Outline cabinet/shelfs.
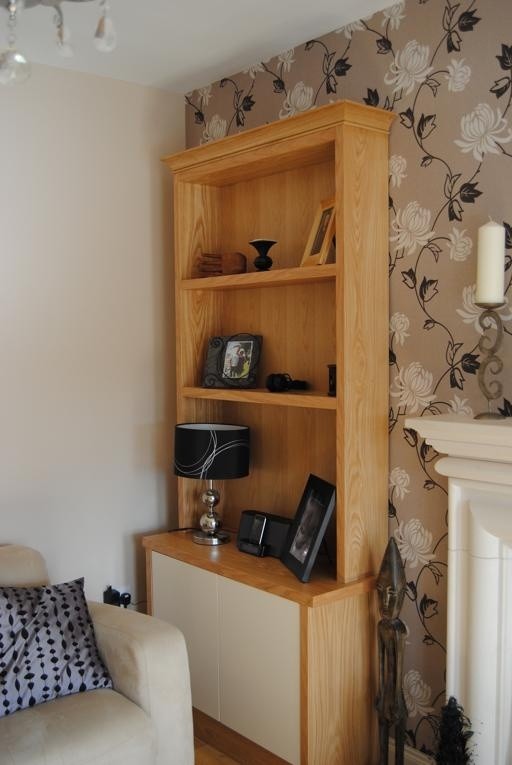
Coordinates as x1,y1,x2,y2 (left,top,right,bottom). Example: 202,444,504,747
140,531,379,765
159,98,399,586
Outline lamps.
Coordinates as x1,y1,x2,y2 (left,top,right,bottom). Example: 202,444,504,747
173,423,249,544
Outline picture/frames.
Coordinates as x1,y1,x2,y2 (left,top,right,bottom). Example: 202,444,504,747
280,474,336,583
298,199,336,265
202,332,262,389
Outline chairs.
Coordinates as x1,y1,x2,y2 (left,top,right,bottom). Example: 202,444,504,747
1,546,194,764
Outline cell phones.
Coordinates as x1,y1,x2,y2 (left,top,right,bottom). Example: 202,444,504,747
250,515,267,546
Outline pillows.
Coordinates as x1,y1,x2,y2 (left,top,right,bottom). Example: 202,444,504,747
0,578,109,719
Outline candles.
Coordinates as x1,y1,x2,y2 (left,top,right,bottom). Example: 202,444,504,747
474,214,506,304
